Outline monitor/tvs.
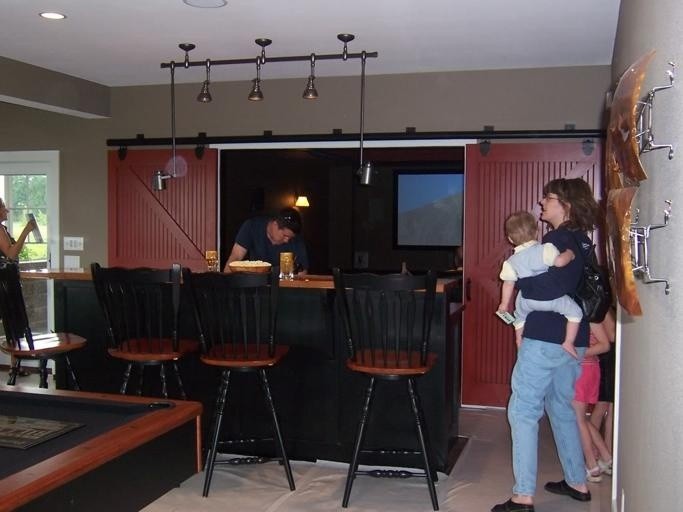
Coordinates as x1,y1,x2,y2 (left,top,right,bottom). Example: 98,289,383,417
1,415,88,450
25,213,43,244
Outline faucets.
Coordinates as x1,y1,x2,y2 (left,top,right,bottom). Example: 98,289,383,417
584,459,613,482
8,367,31,377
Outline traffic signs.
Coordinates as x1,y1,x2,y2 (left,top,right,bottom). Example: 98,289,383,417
63,236,84,251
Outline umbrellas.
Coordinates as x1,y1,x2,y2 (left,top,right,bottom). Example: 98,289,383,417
491,498,535,512
545,480,590,501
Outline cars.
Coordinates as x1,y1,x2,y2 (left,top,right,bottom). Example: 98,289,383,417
281,258,292,280
206,257,217,270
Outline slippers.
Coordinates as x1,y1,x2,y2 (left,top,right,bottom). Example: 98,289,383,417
560,229,613,322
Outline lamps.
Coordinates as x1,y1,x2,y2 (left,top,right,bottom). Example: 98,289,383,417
0,257,88,390
90,261,190,401
330,266,439,511
182,265,295,497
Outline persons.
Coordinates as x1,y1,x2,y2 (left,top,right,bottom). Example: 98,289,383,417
588,299,617,478
0,199,37,378
489,176,610,511
573,309,616,485
495,210,582,358
224,207,311,279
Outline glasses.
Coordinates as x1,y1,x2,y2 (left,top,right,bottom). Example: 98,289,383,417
153,34,379,192
105,124,608,161
295,195,311,208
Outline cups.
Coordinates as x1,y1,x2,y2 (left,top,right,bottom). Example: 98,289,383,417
545,195,559,201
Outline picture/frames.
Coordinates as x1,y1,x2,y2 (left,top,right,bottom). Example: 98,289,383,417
0,384,204,512
